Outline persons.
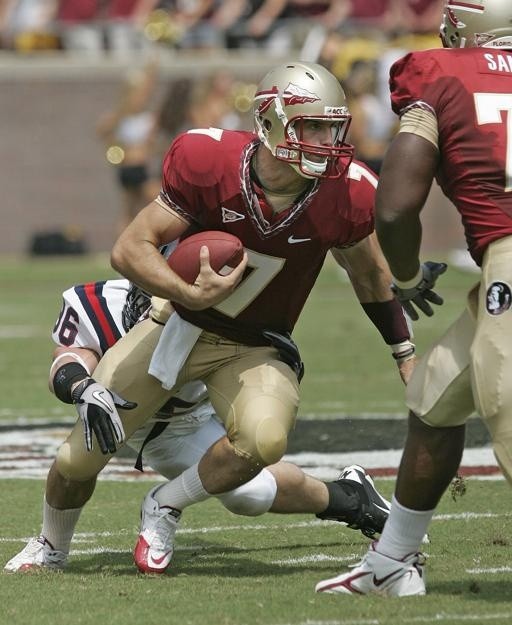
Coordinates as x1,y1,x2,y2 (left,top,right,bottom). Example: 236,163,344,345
311,1,512,598
48,239,394,542
4,59,419,574
1,0,449,227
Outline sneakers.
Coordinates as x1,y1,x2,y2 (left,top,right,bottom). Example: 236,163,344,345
338,465,431,544
3,533,69,577
314,542,428,599
132,481,183,575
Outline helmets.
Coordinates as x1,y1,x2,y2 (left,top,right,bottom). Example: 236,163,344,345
252,60,356,182
438,0,512,51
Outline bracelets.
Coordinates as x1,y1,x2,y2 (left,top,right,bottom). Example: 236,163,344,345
391,345,414,359
388,339,414,368
53,362,91,405
389,262,423,292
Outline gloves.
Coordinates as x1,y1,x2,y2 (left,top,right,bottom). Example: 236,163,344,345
389,259,449,322
69,377,139,454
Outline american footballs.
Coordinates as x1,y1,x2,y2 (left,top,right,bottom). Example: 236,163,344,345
167,230,242,286
